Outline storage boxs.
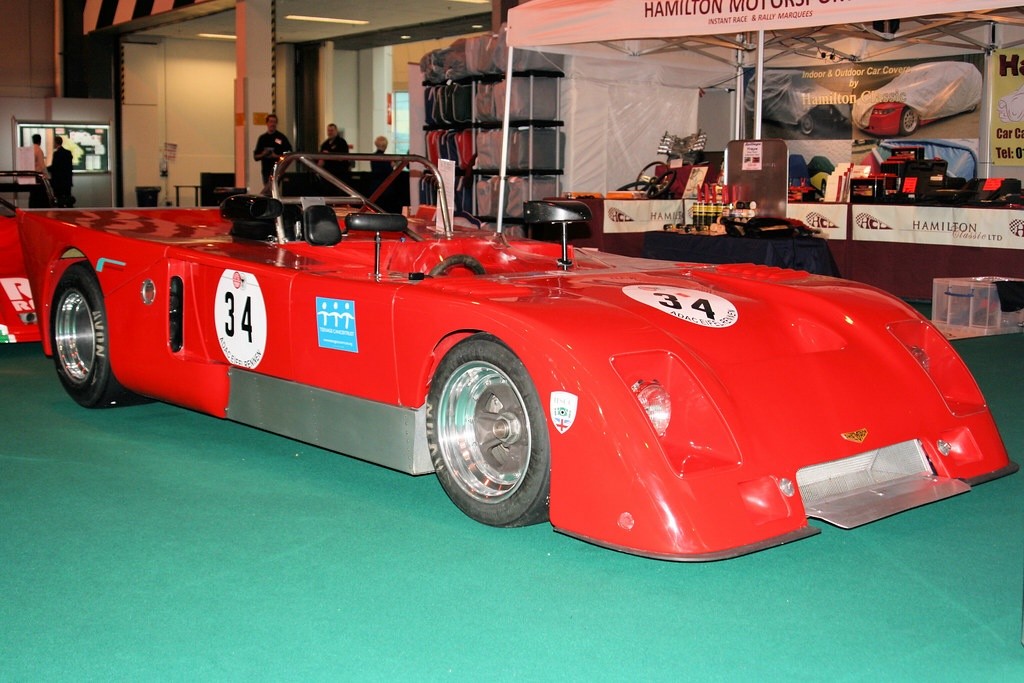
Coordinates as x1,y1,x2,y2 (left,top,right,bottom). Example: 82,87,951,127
933,276,1023,329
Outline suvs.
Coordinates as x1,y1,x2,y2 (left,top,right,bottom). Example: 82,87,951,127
745,70,850,135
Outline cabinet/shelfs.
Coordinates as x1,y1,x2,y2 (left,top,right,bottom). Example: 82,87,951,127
422,71,565,223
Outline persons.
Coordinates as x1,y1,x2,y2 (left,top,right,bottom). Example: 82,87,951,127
370,136,396,185
28,135,48,208
48,136,73,197
320,124,349,176
254,115,292,185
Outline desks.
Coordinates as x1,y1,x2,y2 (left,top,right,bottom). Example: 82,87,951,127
642,231,841,278
173,184,201,209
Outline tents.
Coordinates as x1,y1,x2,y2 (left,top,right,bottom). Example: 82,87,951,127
496,0,1024,236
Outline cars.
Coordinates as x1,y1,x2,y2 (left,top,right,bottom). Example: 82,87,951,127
0,170,65,343
853,59,983,136
14,153,1020,564
